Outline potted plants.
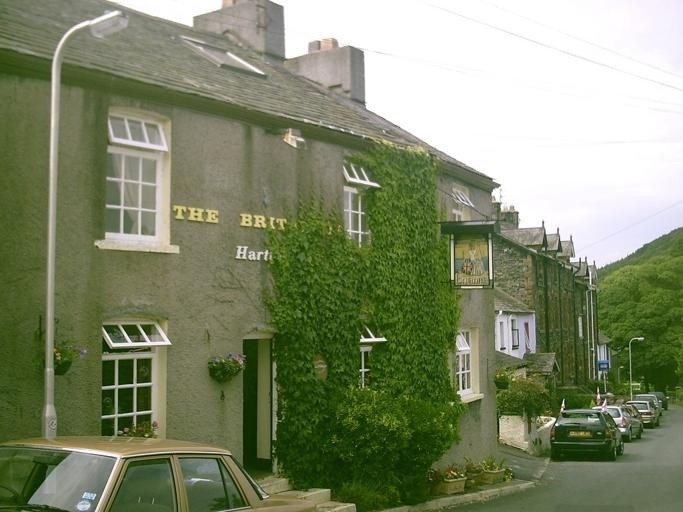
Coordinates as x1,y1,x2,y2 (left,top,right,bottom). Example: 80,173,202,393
464,456,484,483
480,454,506,484
429,466,442,496
439,462,467,495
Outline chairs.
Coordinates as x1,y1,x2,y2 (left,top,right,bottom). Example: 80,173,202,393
31,468,225,512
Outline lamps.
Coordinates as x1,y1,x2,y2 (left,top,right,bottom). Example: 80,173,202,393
265,126,307,151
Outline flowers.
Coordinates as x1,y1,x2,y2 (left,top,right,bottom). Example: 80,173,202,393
116,418,159,438
51,345,87,369
208,353,245,382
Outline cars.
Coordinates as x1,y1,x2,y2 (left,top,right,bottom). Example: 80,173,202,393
0,435,317,512
550,392,670,461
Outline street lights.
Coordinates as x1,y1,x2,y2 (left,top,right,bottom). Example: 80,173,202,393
42,10,129,437
629,337,644,401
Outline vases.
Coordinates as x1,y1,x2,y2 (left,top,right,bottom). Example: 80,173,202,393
42,359,72,376
